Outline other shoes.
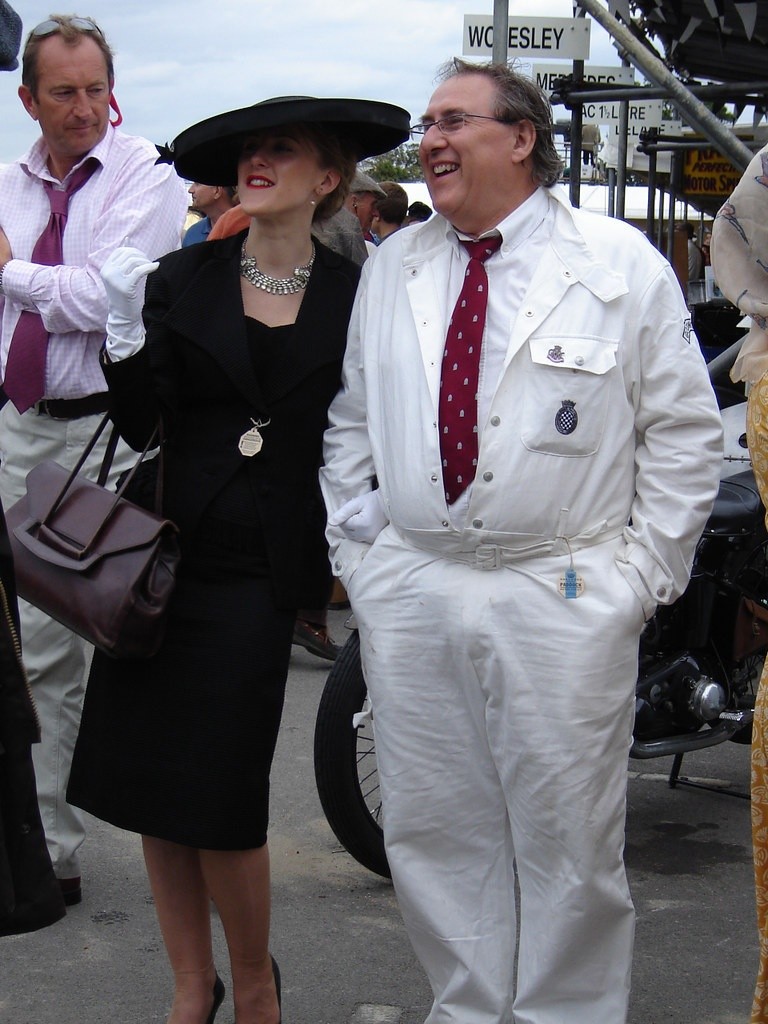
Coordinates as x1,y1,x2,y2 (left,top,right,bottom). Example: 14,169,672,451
57,876,82,905
292,620,344,660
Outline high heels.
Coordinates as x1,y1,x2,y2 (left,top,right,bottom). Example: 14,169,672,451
270,953,282,1024
205,973,225,1024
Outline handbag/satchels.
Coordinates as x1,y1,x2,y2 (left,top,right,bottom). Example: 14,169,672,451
5,398,187,666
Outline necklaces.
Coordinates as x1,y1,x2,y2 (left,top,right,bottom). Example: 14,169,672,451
242,235,314,295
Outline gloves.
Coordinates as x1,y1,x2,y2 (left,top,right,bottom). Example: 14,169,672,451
326,487,389,545
96,236,160,363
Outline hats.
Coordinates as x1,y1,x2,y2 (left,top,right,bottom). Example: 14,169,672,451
350,172,387,201
153,95,412,188
0,0,22,71
675,222,697,238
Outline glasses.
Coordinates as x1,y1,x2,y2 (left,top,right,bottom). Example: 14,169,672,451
22,18,109,85
407,114,518,142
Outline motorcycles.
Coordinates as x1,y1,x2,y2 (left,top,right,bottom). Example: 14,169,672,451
313,299,768,879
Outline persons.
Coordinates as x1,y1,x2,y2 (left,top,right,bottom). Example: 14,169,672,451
57,90,410,1024
0,18,187,905
317,60,725,1024
180,170,432,660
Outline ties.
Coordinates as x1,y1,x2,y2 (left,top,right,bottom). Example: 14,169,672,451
3,157,101,415
438,233,503,506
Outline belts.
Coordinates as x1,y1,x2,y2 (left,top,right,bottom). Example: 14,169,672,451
31,391,110,418
